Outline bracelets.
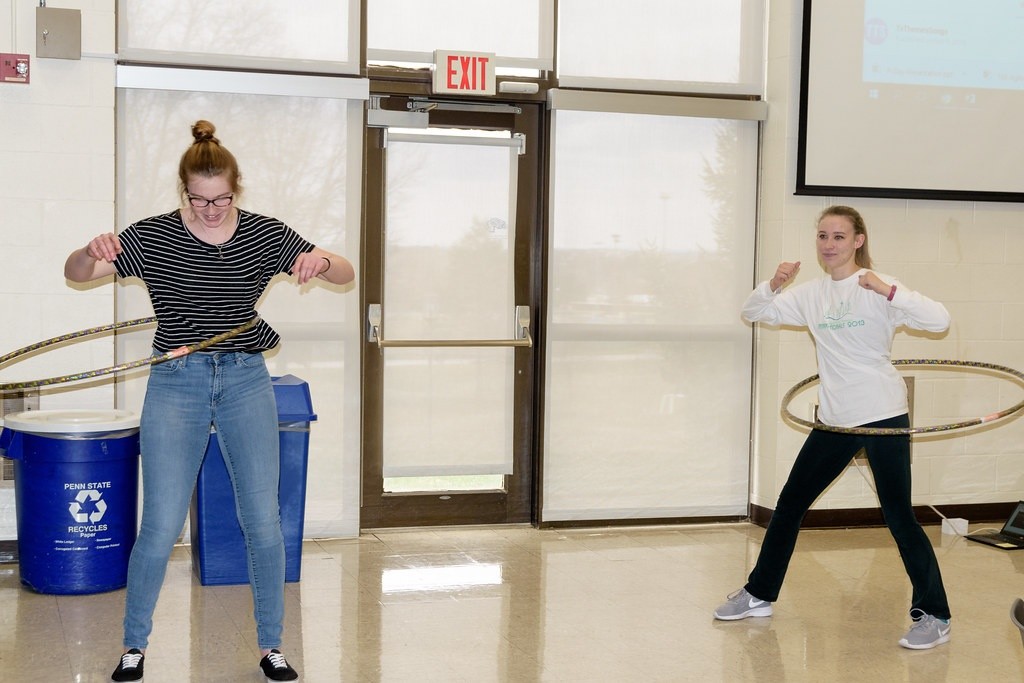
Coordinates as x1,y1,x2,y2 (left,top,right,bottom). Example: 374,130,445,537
318,257,331,274
886,284,897,301
771,281,776,290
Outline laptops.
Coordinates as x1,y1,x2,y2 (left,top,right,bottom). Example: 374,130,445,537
963,501,1024,550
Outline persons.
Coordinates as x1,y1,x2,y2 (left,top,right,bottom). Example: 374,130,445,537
713,207,953,650
65,121,355,682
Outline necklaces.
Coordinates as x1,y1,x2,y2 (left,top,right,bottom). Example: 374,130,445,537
196,218,232,259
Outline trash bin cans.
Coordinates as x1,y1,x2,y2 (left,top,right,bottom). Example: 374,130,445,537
192,371,319,587
0,408,143,596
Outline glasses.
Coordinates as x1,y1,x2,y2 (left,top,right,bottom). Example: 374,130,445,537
186,188,234,207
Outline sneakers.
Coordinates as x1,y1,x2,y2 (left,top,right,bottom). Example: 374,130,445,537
898,608,951,649
111,648,145,683
714,588,773,620
258,649,299,683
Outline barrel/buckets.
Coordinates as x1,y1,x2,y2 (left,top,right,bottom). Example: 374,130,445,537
0,409,140,597
